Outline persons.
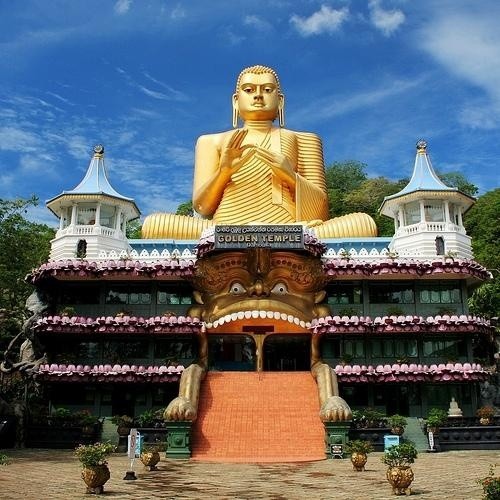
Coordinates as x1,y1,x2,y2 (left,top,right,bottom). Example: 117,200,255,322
140,66,380,240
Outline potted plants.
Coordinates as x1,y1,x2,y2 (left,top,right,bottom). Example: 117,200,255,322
389,414,408,435
73,442,117,494
426,407,447,433
381,444,418,495
478,407,494,425
141,446,160,471
347,439,369,472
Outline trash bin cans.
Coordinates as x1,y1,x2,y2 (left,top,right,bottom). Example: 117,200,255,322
383,435,401,458
128,431,144,458
